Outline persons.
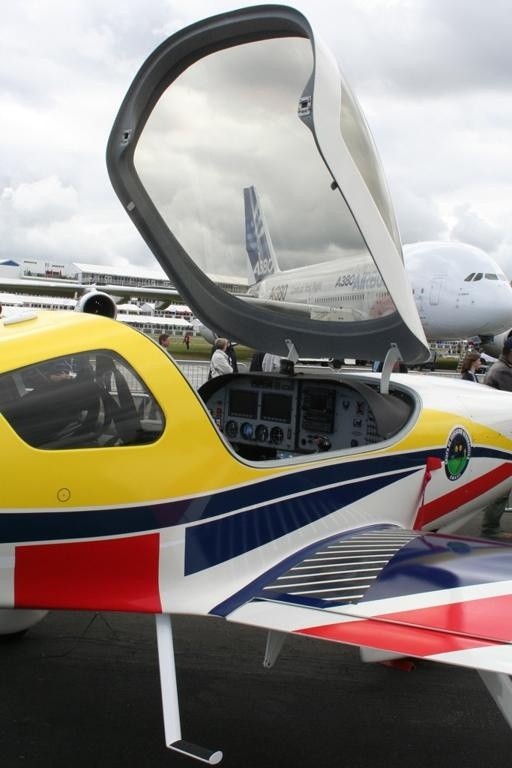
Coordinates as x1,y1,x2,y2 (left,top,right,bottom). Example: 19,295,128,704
96,355,112,391
159,334,265,378
372,330,512,391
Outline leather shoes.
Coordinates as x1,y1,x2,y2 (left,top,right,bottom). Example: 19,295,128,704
480,530,512,539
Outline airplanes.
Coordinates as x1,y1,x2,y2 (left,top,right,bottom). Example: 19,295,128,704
0,184,512,376
0,0,511,768
